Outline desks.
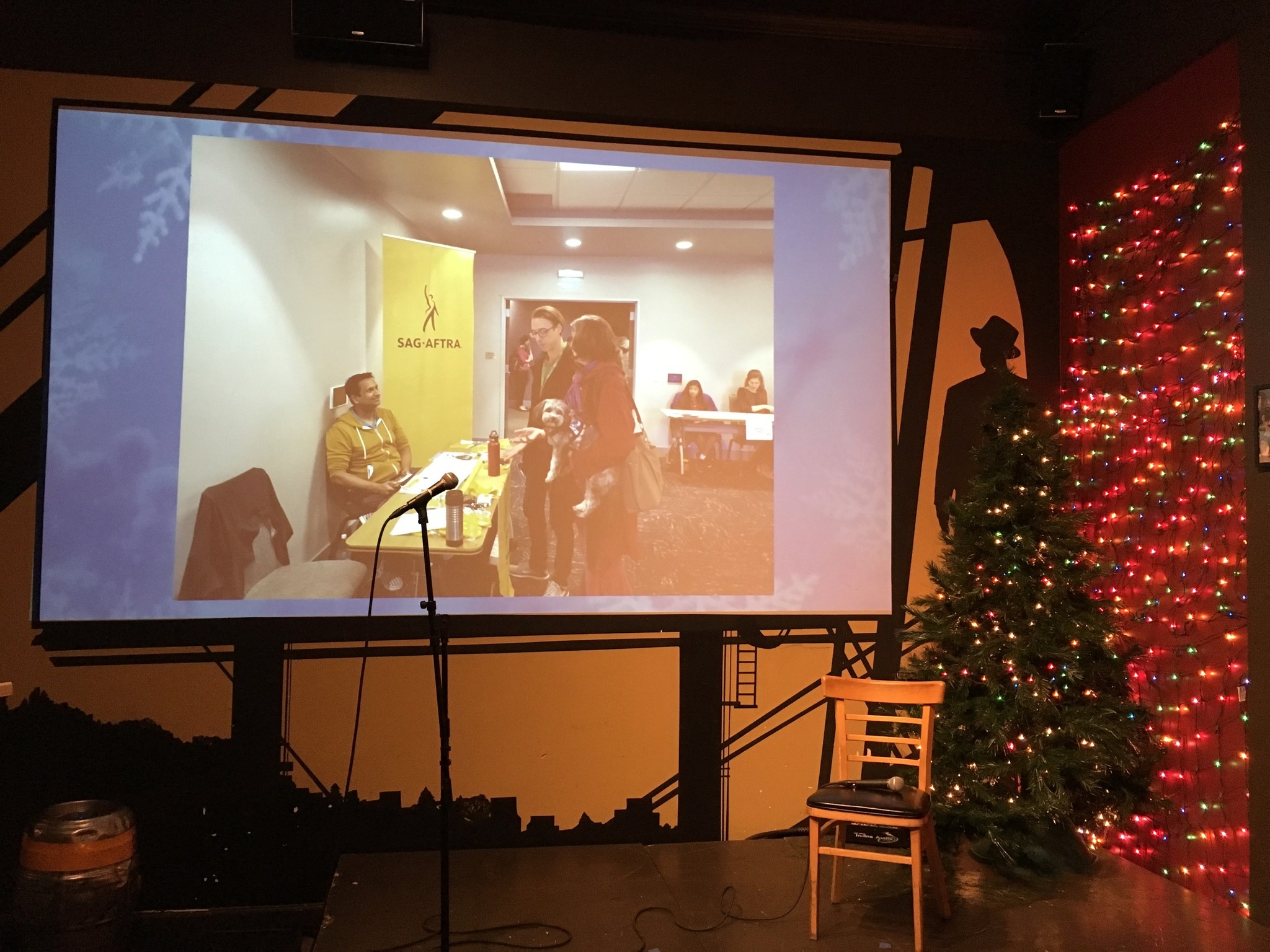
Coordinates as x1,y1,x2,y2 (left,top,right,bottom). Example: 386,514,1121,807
666,407,774,479
346,439,517,596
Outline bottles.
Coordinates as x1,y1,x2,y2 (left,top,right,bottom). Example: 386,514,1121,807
488,430,500,476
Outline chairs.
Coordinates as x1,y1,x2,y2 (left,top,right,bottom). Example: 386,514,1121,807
671,392,753,467
203,470,367,598
805,675,951,952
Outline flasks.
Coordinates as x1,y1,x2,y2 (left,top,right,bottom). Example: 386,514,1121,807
446,490,463,546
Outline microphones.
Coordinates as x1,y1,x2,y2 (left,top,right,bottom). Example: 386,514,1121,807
390,473,458,520
837,776,904,792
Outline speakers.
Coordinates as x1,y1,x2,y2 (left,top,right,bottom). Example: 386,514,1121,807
291,0,425,52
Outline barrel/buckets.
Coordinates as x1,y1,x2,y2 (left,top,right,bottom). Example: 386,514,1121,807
19,799,137,929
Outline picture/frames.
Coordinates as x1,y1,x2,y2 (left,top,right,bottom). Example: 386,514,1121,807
1253,383,1270,471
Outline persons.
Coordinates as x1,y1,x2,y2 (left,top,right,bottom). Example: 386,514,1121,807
613,326,630,378
507,305,577,596
516,335,534,412
510,314,639,596
324,372,416,590
668,379,715,474
736,367,774,480
